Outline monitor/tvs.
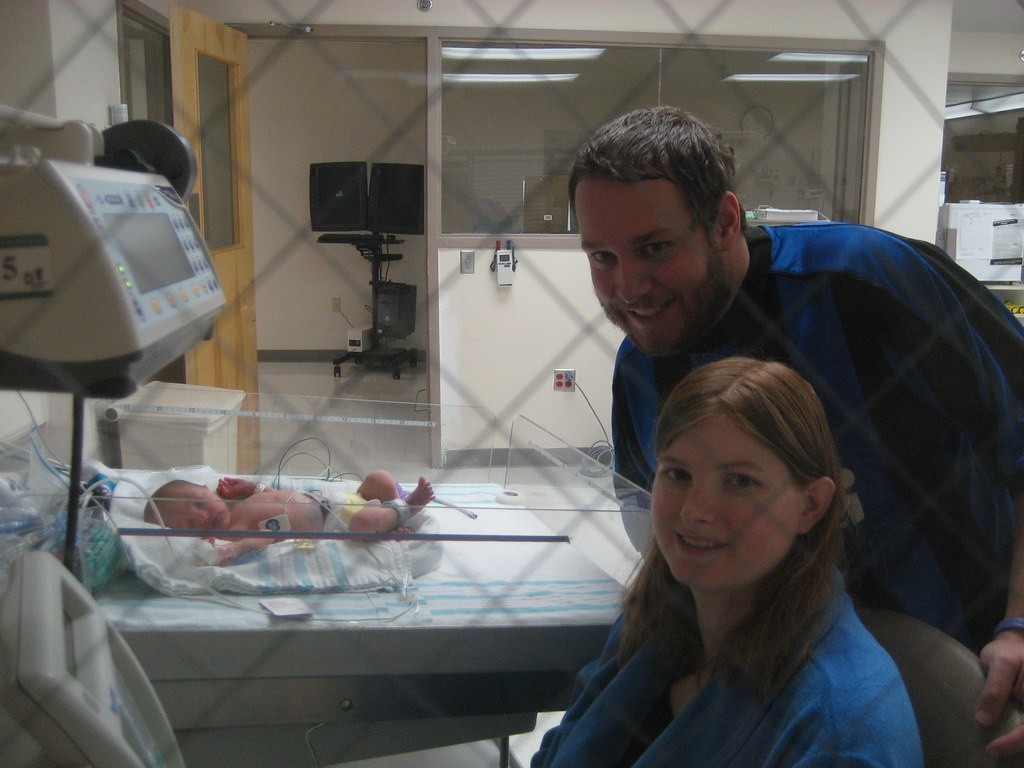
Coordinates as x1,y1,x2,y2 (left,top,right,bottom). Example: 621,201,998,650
309,161,368,232
366,163,425,234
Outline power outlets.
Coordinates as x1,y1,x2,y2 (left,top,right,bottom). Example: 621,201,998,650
554,369,576,392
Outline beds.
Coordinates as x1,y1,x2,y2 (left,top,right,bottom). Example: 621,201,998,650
0,422,655,768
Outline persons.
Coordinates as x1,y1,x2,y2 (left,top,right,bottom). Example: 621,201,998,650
143,470,436,569
525,353,923,768
565,104,1024,755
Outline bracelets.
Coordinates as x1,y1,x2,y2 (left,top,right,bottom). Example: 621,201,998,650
991,615,1024,640
